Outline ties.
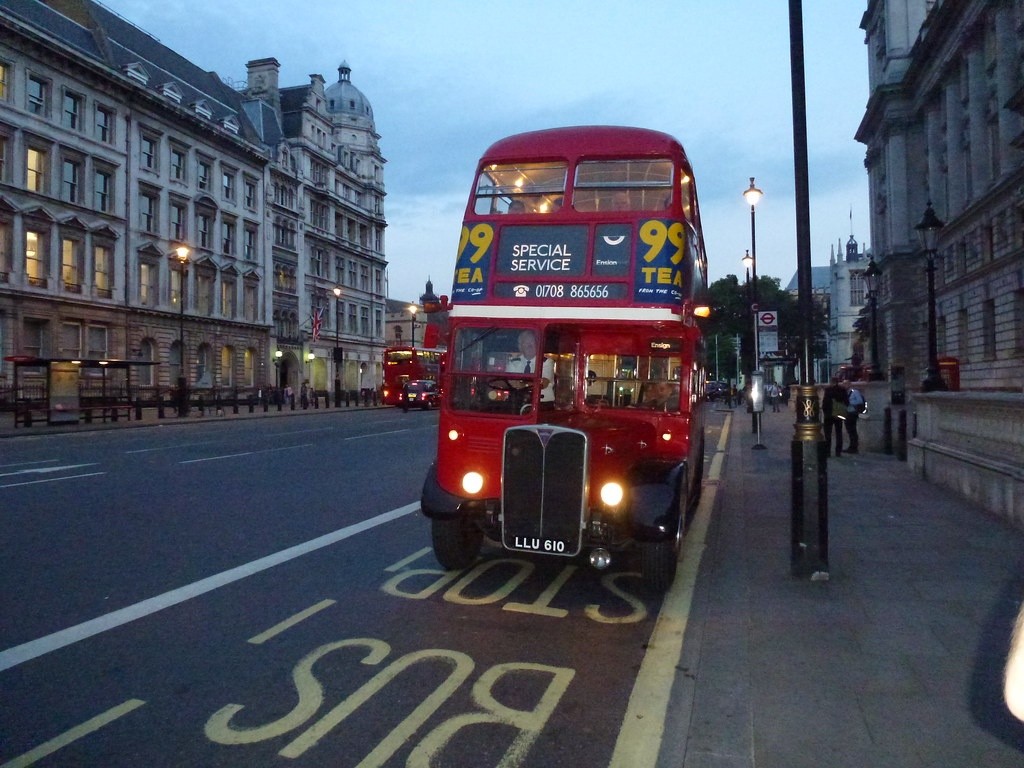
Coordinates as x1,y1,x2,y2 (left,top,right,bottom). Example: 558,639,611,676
524,361,531,374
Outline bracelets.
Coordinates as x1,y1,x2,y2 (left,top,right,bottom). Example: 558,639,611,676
525,381,529,388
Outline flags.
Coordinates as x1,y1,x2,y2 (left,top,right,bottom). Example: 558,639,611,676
310,310,322,342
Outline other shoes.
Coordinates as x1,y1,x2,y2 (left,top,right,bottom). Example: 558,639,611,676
836,452,840,457
842,447,858,454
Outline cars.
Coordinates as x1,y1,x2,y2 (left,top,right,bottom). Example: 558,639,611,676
705,380,730,400
398,380,441,411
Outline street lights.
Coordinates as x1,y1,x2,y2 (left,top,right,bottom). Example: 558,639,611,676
740,177,765,450
333,286,344,408
174,243,190,419
409,301,419,348
861,255,884,381
914,197,949,392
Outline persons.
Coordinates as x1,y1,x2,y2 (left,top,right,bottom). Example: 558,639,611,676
965,571,1024,756
507,201,525,214
626,380,680,411
486,327,557,416
768,383,783,413
300,379,313,406
819,378,850,457
283,383,292,407
612,191,631,210
841,379,864,454
731,383,752,407
551,196,564,212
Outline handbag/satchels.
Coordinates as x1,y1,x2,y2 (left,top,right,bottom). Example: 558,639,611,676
848,389,866,412
831,398,848,421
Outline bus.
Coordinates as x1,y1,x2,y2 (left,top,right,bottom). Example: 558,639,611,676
419,125,724,592
381,345,451,408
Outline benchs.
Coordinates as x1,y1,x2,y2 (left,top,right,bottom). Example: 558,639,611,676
24,402,132,428
